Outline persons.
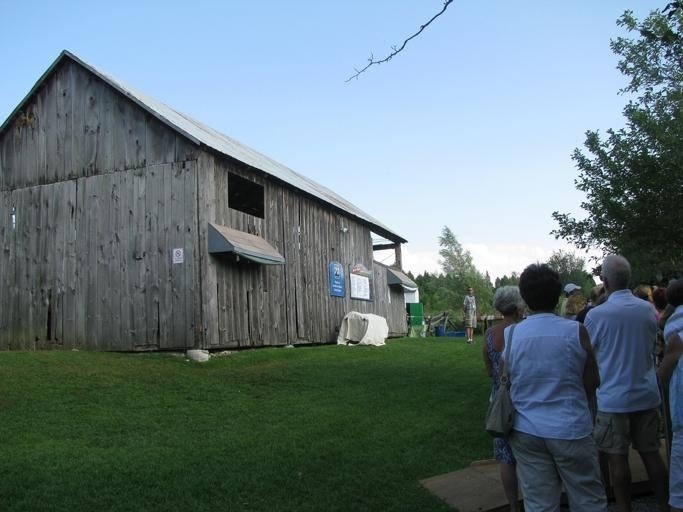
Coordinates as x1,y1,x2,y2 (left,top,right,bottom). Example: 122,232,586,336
463,287,477,345
581,254,670,511
554,277,683,441
480,286,528,512
500,261,611,512
656,277,683,511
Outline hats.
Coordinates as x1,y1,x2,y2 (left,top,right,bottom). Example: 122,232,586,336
563,283,581,298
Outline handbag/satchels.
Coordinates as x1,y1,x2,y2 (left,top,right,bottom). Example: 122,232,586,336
486,383,514,437
